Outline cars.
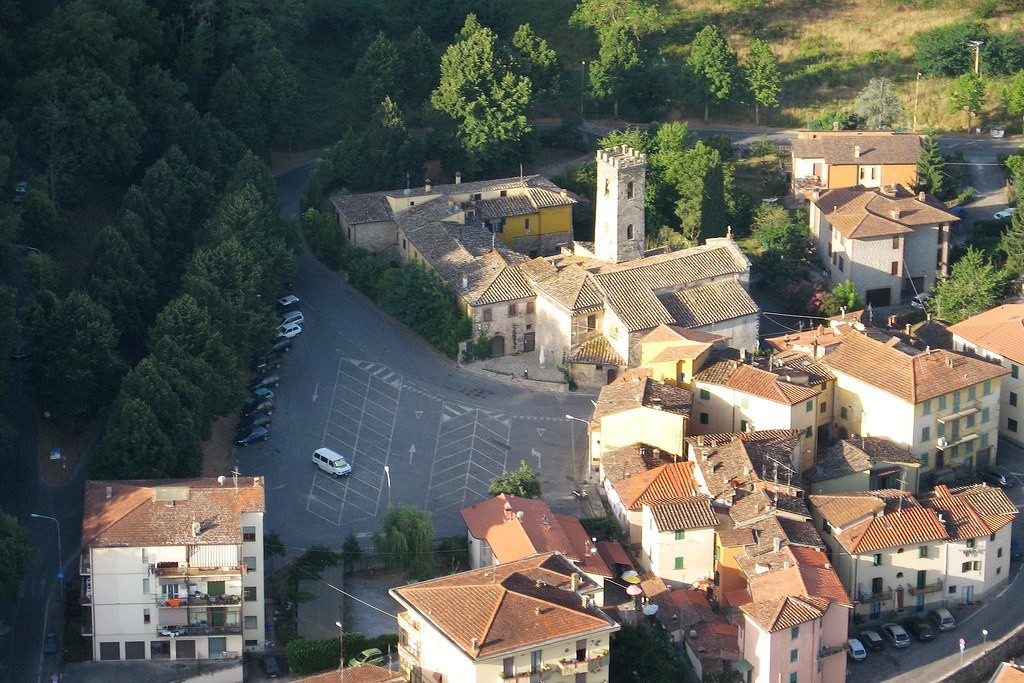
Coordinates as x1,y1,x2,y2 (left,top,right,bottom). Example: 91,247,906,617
348,648,385,671
908,619,936,643
241,335,293,418
949,206,968,220
233,425,270,447
910,292,933,311
276,323,303,341
45,633,58,655
861,630,884,652
880,622,911,649
1011,537,1024,560
993,208,1017,220
236,411,272,432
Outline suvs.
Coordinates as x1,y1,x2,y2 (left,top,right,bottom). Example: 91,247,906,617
276,310,305,328
929,607,956,633
978,466,1015,489
272,295,300,314
847,638,866,663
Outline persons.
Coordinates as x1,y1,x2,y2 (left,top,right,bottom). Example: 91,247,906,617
524,366,528,379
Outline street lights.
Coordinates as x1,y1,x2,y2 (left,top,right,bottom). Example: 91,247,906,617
336,620,344,671
30,512,66,604
566,415,591,476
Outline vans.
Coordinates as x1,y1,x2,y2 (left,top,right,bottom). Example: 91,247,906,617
990,124,1007,139
312,448,352,480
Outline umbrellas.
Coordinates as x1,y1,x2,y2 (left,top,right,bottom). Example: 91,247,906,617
621,570,642,584
626,584,642,595
643,603,659,615
538,344,545,365
